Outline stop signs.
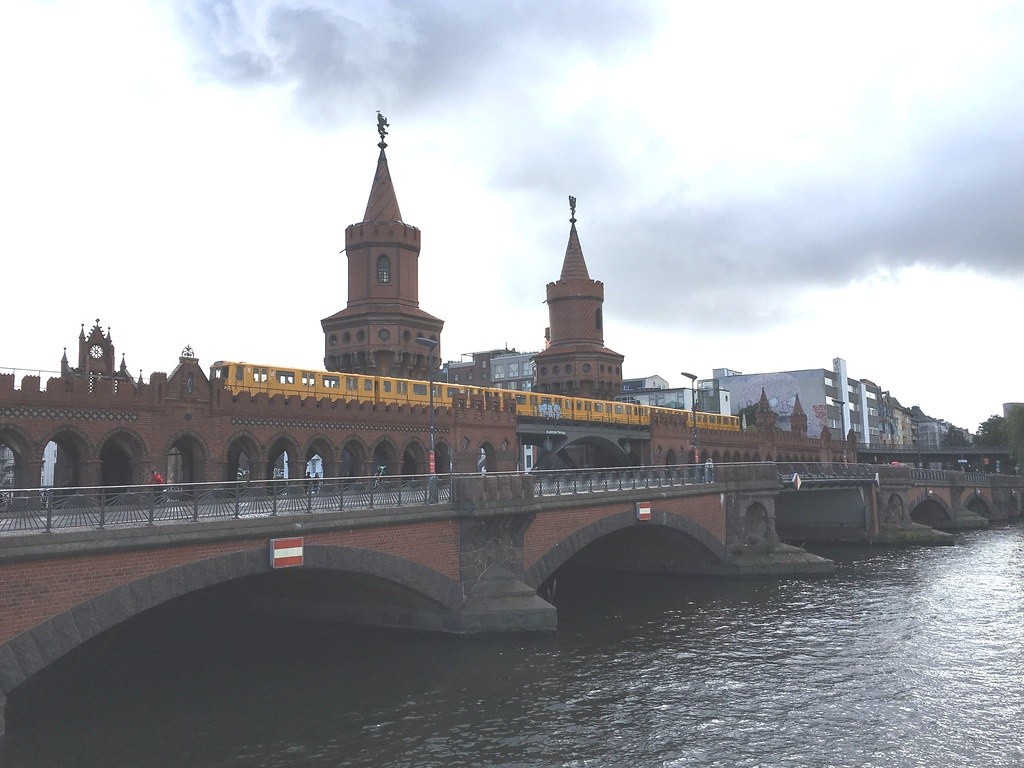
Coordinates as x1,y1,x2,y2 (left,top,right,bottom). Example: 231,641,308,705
270,537,304,569
637,502,652,520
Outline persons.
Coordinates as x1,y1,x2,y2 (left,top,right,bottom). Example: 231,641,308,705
314,473,319,478
305,472,311,478
374,466,385,487
705,458,713,483
146,469,164,504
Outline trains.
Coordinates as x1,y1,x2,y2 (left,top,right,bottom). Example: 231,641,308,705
209,360,741,433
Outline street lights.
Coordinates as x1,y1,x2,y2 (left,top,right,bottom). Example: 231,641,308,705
680,372,700,464
414,338,437,474
831,399,847,462
